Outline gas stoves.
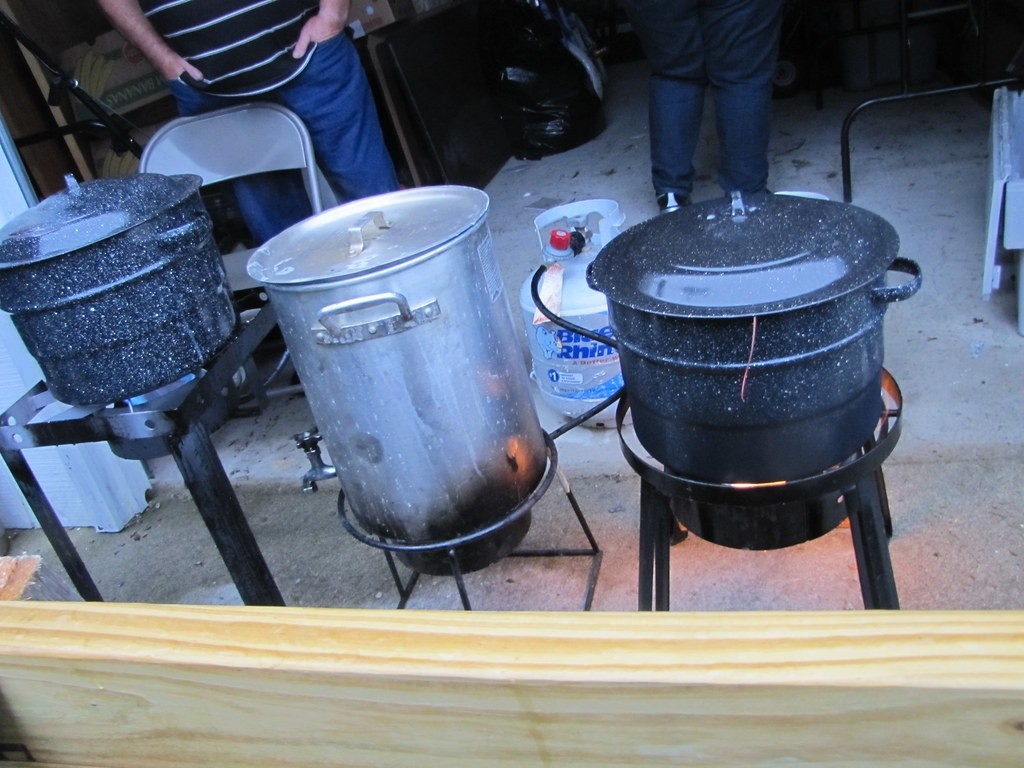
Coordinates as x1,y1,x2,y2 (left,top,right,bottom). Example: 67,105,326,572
0,284,296,606
614,365,904,614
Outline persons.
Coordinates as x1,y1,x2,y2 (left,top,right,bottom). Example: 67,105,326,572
612,1,785,222
100,0,404,397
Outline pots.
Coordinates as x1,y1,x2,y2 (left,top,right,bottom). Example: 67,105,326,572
583,188,924,482
0,172,240,406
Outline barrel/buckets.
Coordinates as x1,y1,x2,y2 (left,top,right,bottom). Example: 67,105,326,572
246,181,553,551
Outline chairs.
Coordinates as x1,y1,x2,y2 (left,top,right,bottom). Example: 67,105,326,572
140,106,335,401
